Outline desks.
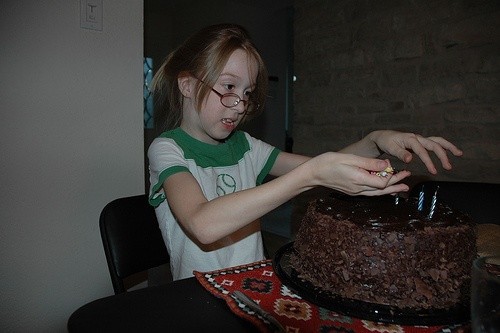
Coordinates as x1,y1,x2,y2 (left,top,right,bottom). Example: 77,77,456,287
67,222,500,333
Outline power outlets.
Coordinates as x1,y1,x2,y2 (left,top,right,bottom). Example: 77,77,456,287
79,0,103,31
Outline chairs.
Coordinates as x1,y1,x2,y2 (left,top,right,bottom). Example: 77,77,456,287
99,194,173,296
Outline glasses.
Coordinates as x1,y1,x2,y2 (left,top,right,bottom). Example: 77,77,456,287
195,75,261,116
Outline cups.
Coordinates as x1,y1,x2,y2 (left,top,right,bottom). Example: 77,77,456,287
471,255,500,333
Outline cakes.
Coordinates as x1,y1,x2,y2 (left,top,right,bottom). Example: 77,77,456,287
286,190,477,311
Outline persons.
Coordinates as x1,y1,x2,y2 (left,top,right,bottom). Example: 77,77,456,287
147,25,462,281
